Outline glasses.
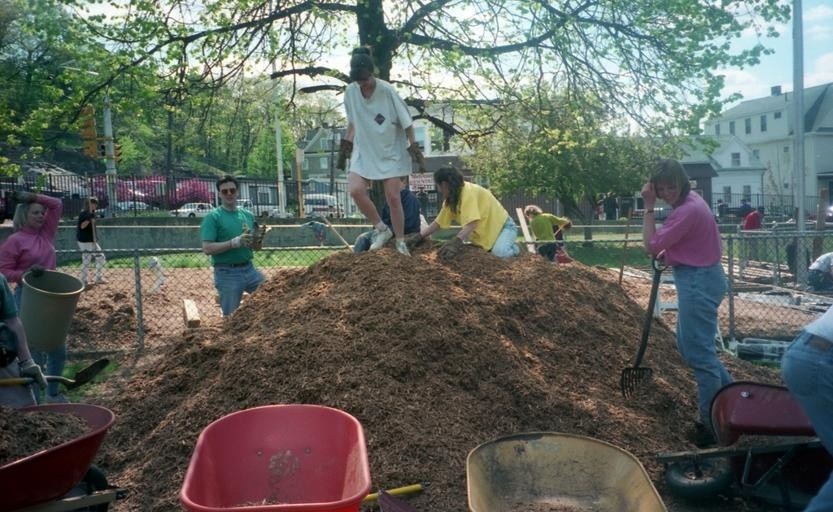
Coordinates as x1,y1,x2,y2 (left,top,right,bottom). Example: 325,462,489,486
221,188,236,195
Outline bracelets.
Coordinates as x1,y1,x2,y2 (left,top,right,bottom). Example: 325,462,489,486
643,208,654,215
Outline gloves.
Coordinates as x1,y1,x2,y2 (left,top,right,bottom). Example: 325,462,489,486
335,139,353,170
19,358,48,390
436,236,463,260
407,142,425,173
28,263,44,276
406,232,422,249
253,221,266,250
5,191,36,203
231,229,255,248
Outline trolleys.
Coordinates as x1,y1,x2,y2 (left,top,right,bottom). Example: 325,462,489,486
1,403,119,510
463,430,673,512
177,402,371,512
652,381,831,509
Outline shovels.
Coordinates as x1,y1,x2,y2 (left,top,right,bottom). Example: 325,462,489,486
621,255,668,404
1,356,110,391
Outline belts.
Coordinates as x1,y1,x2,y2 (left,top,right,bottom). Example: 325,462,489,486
810,335,832,350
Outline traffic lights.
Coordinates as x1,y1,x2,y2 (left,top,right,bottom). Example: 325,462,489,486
114,143,122,163
100,143,105,157
78,102,96,157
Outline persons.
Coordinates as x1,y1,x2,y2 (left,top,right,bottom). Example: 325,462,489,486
785,234,810,276
407,166,521,258
782,303,833,512
524,205,570,261
353,177,421,254
0,187,69,403
596,192,619,221
77,197,109,287
534,216,572,250
808,250,833,292
0,272,48,407
641,159,733,431
336,47,425,256
718,197,765,261
200,175,265,316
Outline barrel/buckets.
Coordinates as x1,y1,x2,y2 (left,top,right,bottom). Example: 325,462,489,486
18,271,85,355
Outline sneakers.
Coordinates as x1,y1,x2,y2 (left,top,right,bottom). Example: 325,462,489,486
370,226,393,250
395,240,411,257
47,393,70,402
96,280,107,283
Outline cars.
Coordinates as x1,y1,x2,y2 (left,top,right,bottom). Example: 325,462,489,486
632,202,832,225
